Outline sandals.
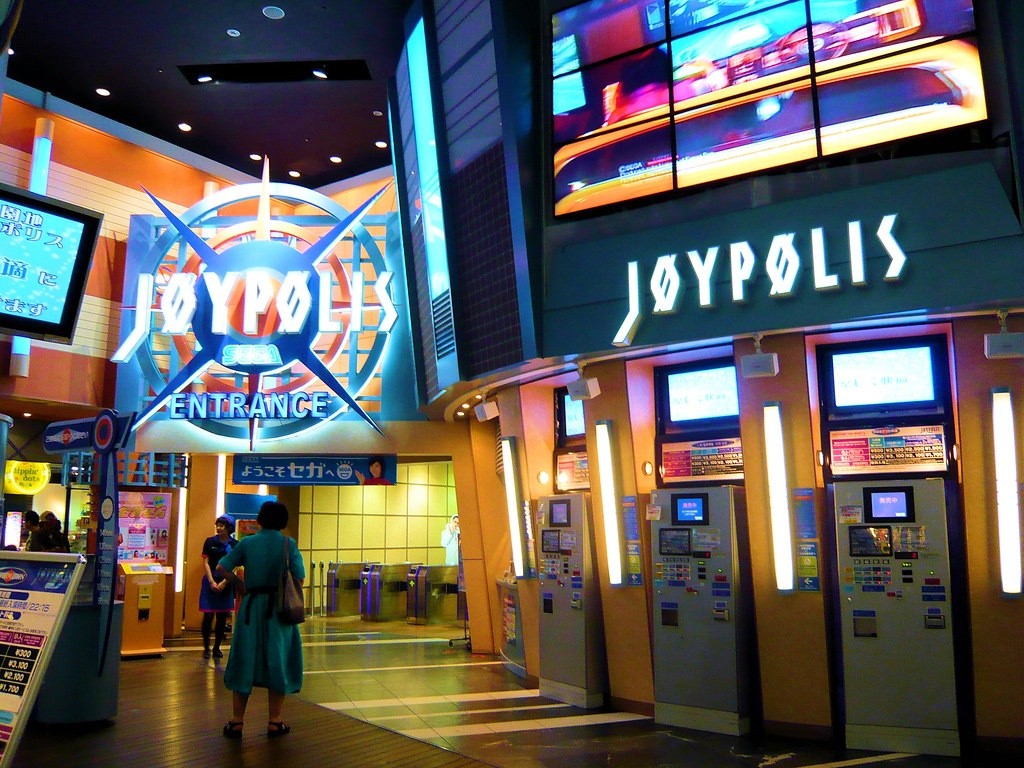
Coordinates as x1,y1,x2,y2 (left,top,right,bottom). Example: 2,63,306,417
266,721,290,737
223,721,243,738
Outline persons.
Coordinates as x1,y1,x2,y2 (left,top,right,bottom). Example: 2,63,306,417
216,500,306,743
197,513,240,659
441,515,461,566
21,510,72,553
354,457,394,484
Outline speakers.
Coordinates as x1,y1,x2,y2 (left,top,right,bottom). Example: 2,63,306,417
474,400,500,423
567,377,601,401
742,353,780,378
984,332,1024,359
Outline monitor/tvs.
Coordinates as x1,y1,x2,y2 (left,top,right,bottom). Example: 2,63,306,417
829,345,943,412
664,363,739,425
870,492,907,517
562,394,586,437
0,183,105,346
552,503,567,523
676,499,703,520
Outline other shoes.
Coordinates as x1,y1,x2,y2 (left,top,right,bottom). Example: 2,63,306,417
213,649,223,657
204,650,210,658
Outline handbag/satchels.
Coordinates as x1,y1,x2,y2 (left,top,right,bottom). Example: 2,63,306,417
277,541,305,624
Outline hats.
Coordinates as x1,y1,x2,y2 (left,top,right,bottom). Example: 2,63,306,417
219,514,235,535
39,511,57,522
